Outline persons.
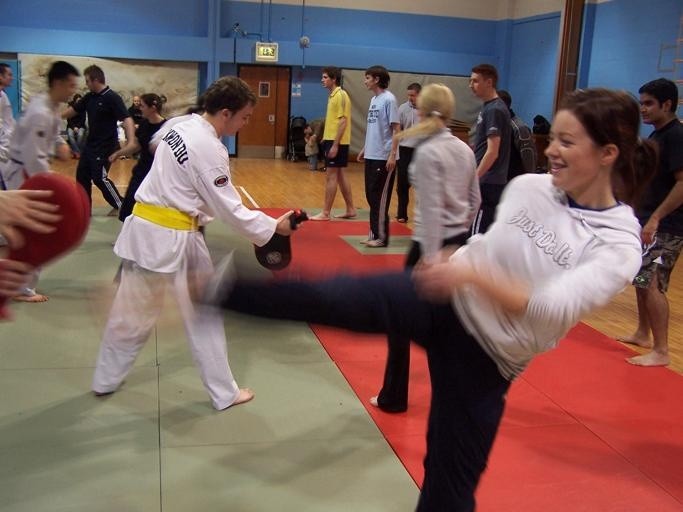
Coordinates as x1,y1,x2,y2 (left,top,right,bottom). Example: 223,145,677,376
304,64,535,244
91,76,305,413
369,82,482,412
617,78,683,367
186,87,661,512
0,59,170,323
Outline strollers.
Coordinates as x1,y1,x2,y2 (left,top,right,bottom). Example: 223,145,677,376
285,115,309,162
